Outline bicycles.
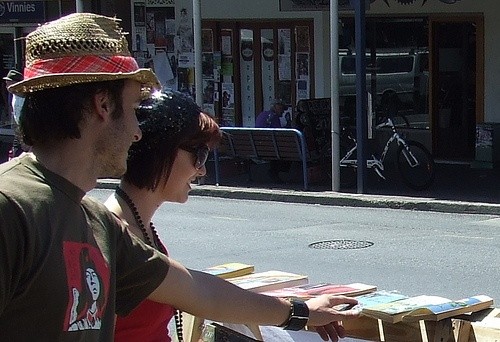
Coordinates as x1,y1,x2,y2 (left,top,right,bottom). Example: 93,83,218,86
340,114,435,191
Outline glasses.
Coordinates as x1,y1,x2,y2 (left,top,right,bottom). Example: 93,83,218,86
178,144,210,169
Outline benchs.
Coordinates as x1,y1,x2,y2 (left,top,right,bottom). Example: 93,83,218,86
214,127,316,190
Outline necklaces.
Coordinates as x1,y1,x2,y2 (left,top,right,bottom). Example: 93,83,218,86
113,184,186,341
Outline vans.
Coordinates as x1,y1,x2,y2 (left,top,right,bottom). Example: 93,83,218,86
338,48,429,114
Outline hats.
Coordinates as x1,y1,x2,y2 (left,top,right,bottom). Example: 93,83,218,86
3,70,24,82
126,88,201,161
270,98,288,110
7,12,162,98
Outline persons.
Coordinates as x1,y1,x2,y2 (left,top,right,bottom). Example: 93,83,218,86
3,70,28,160
254,102,288,129
0,11,362,341
96,88,226,342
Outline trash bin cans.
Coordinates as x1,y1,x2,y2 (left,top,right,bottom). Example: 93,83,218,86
0,124,15,164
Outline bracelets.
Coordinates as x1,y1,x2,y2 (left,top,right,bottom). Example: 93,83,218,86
277,297,311,333
